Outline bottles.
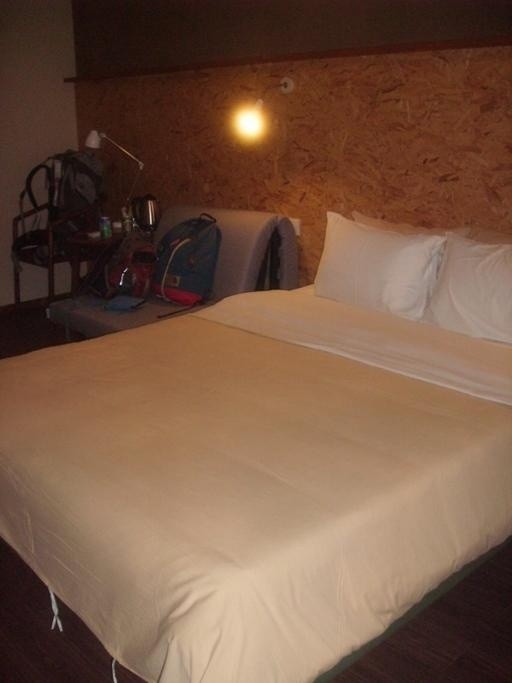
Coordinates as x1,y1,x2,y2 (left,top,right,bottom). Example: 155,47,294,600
121,195,133,230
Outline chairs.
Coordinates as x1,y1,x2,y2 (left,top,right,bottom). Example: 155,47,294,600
11,148,100,314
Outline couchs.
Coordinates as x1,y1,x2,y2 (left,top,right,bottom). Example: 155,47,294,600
48,205,300,339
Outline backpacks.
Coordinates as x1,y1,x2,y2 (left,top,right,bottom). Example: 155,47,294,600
24,148,103,232
79,213,222,318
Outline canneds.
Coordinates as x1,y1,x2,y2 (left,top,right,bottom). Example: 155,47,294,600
122,216,133,238
100,217,112,239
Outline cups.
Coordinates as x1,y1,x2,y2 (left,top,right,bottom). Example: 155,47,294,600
132,250,157,294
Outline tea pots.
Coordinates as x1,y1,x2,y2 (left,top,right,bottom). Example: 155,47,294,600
134,194,160,230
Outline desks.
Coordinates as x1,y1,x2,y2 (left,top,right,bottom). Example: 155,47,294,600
62,227,122,294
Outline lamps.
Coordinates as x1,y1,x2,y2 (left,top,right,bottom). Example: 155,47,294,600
232,74,298,144
83,127,144,230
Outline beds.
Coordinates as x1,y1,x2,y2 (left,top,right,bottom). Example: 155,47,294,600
0,281,512,683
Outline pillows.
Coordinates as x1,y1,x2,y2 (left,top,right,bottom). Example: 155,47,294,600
349,207,466,238
310,209,445,323
439,229,512,345
469,224,512,244
